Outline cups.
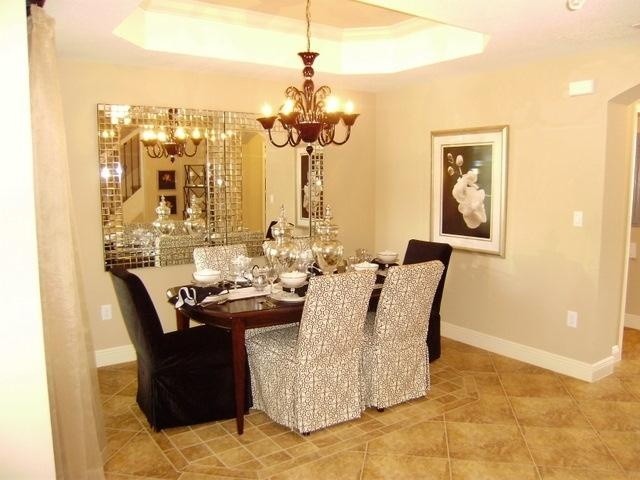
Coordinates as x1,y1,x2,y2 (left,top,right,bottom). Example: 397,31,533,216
346,248,375,266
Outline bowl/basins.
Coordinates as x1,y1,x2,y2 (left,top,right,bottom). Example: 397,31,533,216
193,269,221,283
355,262,379,272
279,272,308,286
378,252,399,261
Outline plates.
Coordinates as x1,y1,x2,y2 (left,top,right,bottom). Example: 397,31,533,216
375,259,400,265
271,292,306,304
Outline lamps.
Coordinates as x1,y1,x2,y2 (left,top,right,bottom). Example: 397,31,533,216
140,120,205,164
258,1,360,152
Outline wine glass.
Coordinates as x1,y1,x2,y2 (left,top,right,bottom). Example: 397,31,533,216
229,255,277,293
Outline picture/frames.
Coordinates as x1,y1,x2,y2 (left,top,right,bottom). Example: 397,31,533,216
156,169,178,215
294,147,323,227
428,123,510,259
